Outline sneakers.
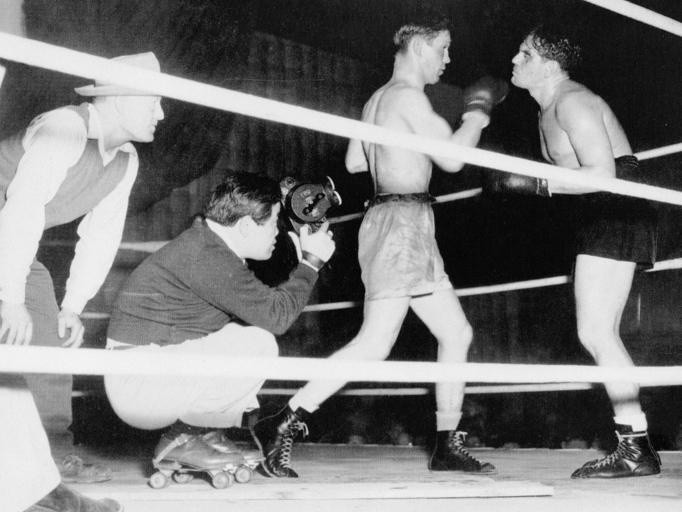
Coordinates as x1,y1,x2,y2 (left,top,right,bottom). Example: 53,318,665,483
249,405,300,477
571,422,661,479
428,430,497,475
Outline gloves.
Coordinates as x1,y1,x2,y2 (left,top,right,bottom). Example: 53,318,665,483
460,76,510,127
478,171,551,208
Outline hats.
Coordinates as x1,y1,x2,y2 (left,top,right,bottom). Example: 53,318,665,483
73,51,160,98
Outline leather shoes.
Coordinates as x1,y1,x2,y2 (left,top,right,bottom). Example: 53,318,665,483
29,483,123,511
55,457,112,483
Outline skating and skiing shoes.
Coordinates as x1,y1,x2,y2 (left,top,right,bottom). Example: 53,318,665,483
149,435,244,489
198,430,266,482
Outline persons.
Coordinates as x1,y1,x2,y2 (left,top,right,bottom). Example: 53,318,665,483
250,6,509,481
0,51,166,488
483,23,663,477
102,172,336,485
0,366,116,510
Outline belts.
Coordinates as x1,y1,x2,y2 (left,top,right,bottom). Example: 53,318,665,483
377,191,436,202
